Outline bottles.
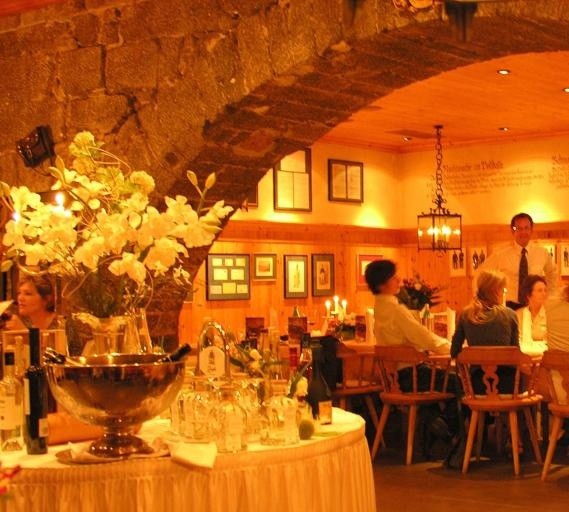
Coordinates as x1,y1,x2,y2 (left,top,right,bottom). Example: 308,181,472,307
307,340,333,426
196,320,310,379
293,264,300,288
355,309,376,343
151,342,193,363
44,344,85,366
422,303,433,333
320,263,325,283
292,305,300,317
168,375,314,455
0,339,46,455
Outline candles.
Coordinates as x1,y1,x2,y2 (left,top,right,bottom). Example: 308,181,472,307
325,301,330,319
342,300,347,315
333,295,339,312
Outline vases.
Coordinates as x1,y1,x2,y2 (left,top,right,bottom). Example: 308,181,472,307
71,312,154,357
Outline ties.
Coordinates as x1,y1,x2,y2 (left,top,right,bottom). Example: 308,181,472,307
518,248,528,302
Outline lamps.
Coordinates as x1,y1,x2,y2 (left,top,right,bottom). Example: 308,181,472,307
416,125,463,252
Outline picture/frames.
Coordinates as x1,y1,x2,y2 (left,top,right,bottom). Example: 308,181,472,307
328,158,363,202
274,146,312,211
205,253,250,301
253,253,278,279
248,186,258,207
311,253,335,297
283,254,308,299
356,252,385,287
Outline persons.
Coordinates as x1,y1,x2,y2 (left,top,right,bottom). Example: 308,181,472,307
365,260,467,457
450,213,569,460
1,275,67,361
319,265,326,282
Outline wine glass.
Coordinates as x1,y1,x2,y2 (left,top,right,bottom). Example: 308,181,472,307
43,352,187,459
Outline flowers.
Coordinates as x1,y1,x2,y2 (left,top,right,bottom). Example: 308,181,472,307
0,128,235,357
393,269,445,310
